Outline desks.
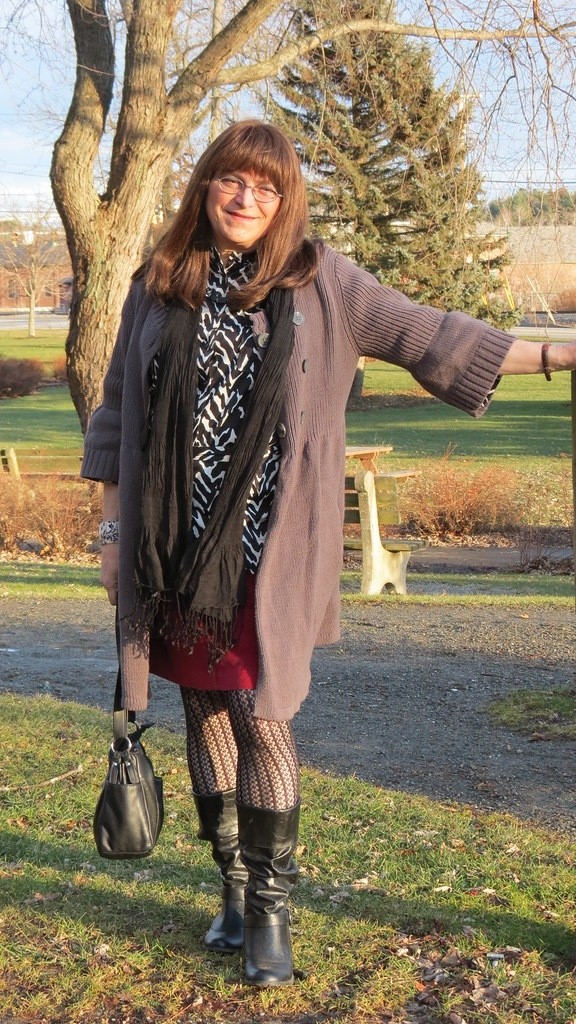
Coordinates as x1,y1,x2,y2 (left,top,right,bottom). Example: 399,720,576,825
345,446,393,474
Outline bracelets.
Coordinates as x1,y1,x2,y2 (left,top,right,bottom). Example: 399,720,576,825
98,521,119,545
542,343,552,381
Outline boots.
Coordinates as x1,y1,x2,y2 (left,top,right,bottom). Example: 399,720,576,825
191,788,249,953
233,795,302,985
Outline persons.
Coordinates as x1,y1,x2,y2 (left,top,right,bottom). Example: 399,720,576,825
79,120,576,987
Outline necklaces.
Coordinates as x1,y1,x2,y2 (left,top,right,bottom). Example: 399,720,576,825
228,272,244,286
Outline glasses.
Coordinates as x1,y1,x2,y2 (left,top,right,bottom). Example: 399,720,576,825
212,176,284,203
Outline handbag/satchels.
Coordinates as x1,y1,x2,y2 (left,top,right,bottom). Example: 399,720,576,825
93,590,164,860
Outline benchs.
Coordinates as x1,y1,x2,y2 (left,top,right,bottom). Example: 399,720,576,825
0,445,100,498
341,470,422,595
